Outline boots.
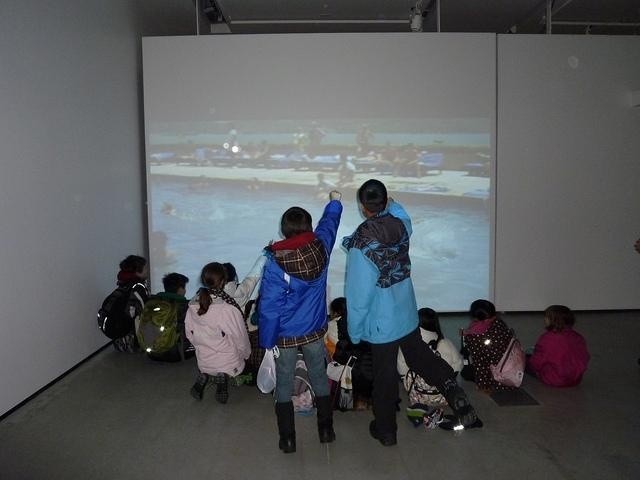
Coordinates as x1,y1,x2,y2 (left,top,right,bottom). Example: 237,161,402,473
437,375,482,430
275,400,296,453
370,393,397,445
315,396,335,442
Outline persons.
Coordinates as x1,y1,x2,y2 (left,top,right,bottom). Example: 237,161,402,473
323,296,346,364
397,299,515,426
524,305,589,387
257,192,344,453
339,180,484,447
111,240,275,403
153,119,427,193
461,144,486,174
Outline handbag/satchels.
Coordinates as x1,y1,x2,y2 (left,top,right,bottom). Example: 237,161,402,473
327,361,353,411
490,337,527,387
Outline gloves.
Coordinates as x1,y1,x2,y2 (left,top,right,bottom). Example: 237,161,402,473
424,407,443,429
406,403,428,426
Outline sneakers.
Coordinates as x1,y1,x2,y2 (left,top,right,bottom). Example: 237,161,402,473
215,373,230,404
191,373,212,400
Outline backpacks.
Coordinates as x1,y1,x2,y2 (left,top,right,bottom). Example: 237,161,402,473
401,340,449,407
137,291,183,353
273,351,318,416
97,282,146,339
